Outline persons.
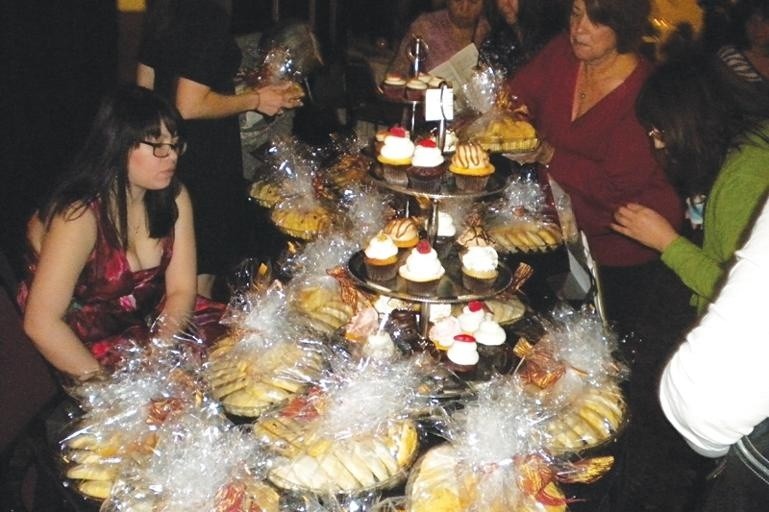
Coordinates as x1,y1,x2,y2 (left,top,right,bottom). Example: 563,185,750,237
14,85,236,384
235,17,324,272
135,0,305,300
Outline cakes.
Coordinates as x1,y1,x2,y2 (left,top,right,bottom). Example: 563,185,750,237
381,70,446,101
363,210,507,382
374,123,496,194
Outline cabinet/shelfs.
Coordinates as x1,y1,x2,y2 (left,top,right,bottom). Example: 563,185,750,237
346,31,523,402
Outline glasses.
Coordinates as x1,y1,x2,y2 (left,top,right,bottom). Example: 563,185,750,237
142,139,187,158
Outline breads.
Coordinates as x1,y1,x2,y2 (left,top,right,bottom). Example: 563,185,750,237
66,435,117,499
207,345,398,512
555,388,624,449
492,222,563,254
483,121,536,144
314,301,354,329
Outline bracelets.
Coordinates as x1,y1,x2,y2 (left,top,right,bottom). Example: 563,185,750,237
254,90,261,110
